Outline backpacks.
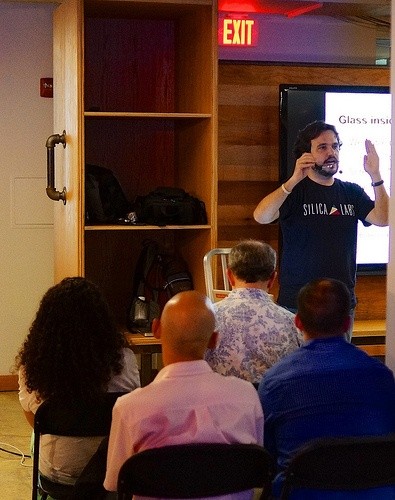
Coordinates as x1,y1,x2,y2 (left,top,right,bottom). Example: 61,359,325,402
125,237,191,331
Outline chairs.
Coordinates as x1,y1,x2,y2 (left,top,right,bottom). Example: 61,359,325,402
117,443,277,500
203,248,273,304
32,393,121,500
281,434,395,500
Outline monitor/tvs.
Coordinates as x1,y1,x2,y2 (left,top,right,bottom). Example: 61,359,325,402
278,84,392,276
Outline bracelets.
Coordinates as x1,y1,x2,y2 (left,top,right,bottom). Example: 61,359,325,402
280,184,290,194
370,180,383,187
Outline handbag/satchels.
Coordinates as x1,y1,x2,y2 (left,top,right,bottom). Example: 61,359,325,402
84,163,128,223
135,185,207,225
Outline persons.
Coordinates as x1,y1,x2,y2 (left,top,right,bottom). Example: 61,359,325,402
16,276,140,491
253,121,389,317
103,290,264,500
204,242,307,389
257,277,395,500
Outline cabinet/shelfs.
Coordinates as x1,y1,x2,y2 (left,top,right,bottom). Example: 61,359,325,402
46,0,219,356
